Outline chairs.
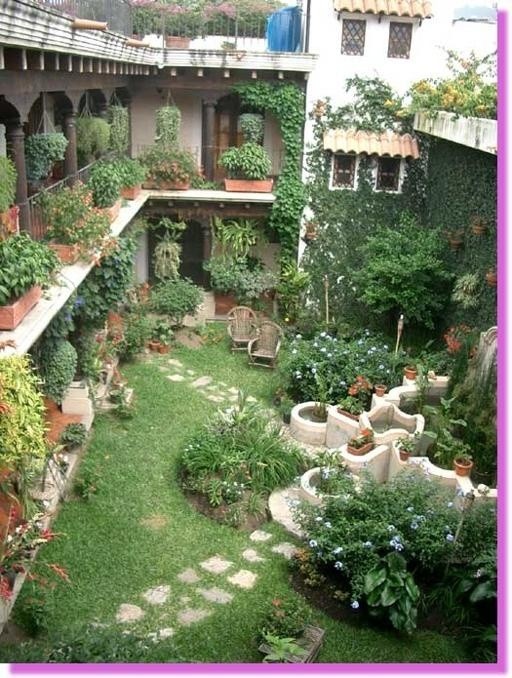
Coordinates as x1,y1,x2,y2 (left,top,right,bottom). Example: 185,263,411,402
225,306,283,372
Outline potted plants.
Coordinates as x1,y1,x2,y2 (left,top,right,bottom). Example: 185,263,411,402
132,1,236,52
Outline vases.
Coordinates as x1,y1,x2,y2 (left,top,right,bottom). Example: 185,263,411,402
109,389,121,402
